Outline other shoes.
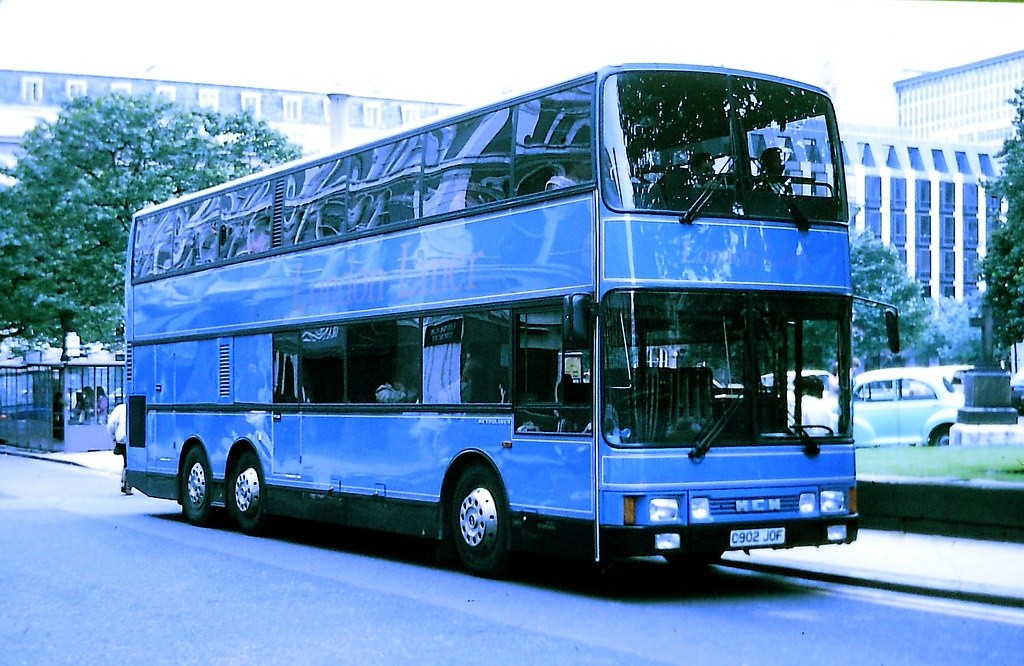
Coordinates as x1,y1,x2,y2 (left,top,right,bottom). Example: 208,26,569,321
121,484,133,495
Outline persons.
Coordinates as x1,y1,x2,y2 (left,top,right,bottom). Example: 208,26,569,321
52,385,109,441
461,341,495,404
828,356,861,438
374,358,419,404
671,153,717,212
750,147,794,196
106,403,135,495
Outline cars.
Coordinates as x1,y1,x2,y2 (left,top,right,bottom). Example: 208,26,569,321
848,367,973,445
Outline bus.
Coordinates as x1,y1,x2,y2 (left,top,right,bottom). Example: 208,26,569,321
122,61,904,576
